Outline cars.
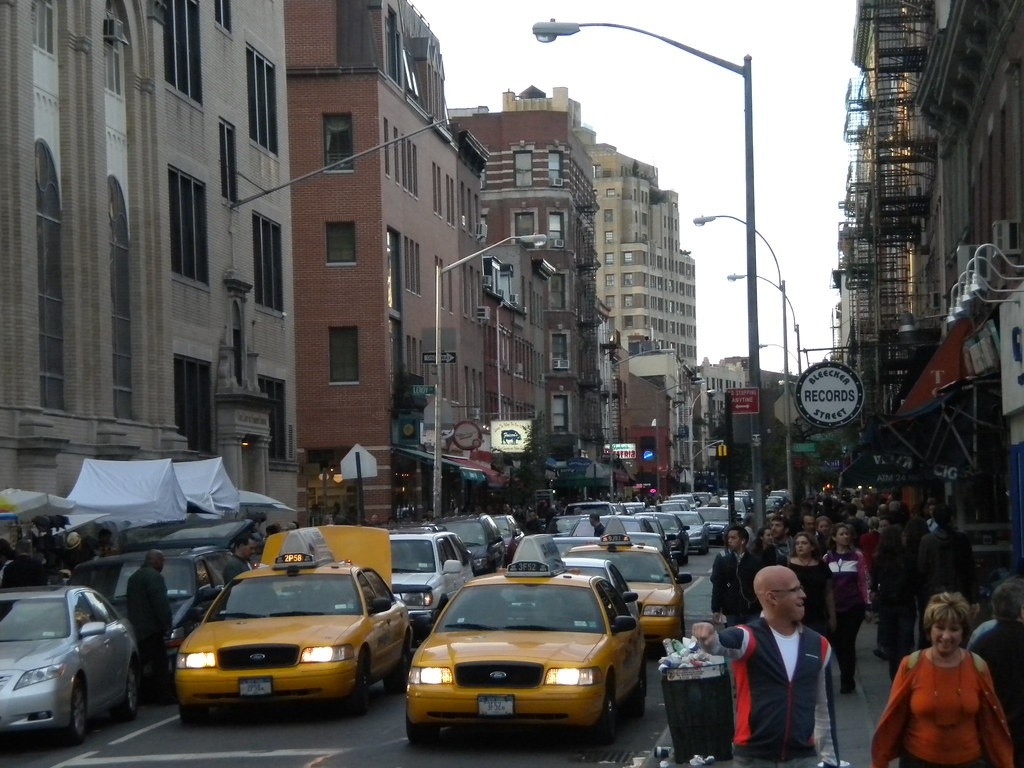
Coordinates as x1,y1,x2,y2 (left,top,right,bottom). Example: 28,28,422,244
547,489,789,575
175,524,409,726
72,518,261,696
562,532,692,651
422,514,524,578
405,533,647,749
502,558,640,625
0,585,141,745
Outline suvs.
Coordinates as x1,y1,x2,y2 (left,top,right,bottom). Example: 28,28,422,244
389,532,474,629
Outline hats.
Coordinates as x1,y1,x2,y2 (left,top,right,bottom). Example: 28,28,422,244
63,532,82,550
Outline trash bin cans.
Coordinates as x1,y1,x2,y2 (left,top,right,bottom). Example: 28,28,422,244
659,662,732,763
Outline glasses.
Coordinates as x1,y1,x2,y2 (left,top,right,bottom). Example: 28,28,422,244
769,584,804,593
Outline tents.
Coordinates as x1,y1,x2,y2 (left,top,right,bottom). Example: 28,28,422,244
0,458,297,570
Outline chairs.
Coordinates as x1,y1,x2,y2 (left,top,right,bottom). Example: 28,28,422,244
247,587,278,611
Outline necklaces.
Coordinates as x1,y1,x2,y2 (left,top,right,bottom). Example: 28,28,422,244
799,557,812,567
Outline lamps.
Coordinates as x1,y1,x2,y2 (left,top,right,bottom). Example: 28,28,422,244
897,311,949,332
946,243,1024,324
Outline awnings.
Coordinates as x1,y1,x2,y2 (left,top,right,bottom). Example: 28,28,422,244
839,442,920,490
886,314,987,428
394,448,610,488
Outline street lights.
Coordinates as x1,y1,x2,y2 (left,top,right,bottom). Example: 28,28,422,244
690,389,716,495
691,214,804,507
656,380,717,502
608,347,677,501
726,273,803,379
431,232,547,521
532,20,767,536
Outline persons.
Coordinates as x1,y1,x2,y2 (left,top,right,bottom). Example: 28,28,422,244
126,549,173,702
871,593,1013,768
589,512,605,537
692,565,839,768
966,576,1024,768
709,489,981,695
223,523,300,586
589,493,670,509
321,499,565,533
0,532,117,589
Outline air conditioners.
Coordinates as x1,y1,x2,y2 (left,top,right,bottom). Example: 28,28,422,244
956,245,991,283
103,18,124,42
477,306,491,319
991,219,1021,258
510,294,518,303
551,239,564,247
483,275,491,286
496,289,504,298
476,223,488,237
553,360,569,368
551,178,563,186
